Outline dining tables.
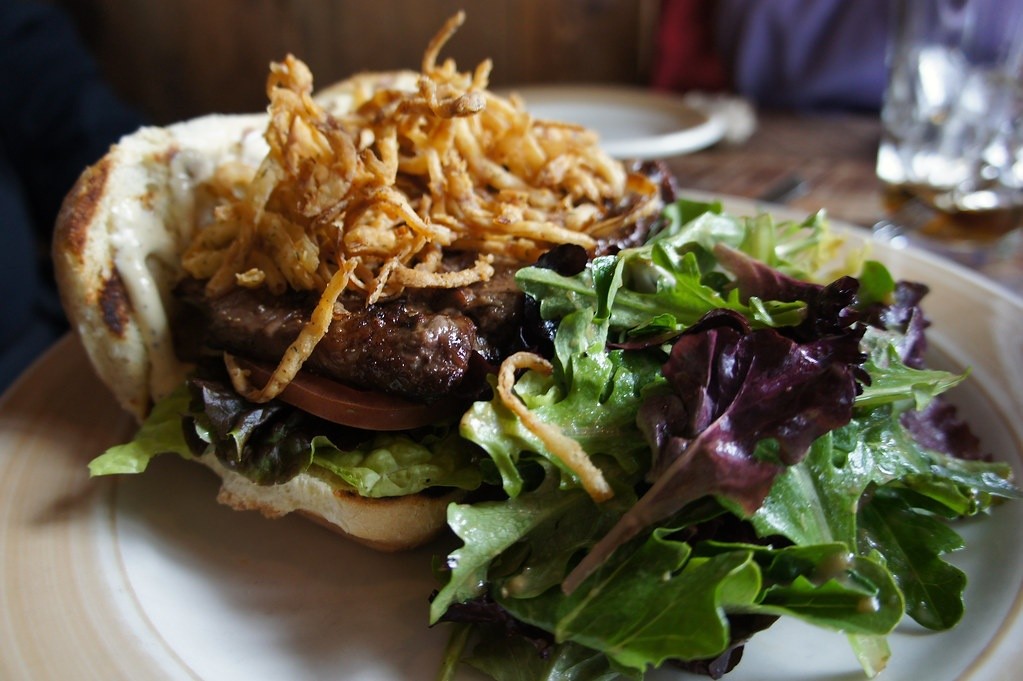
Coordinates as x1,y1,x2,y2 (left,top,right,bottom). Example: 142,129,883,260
625,116,1022,302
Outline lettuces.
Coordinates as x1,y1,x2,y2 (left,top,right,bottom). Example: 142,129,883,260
88,202,1022,681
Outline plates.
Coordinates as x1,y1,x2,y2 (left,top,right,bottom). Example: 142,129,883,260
487,81,724,158
0,188,1022,681
